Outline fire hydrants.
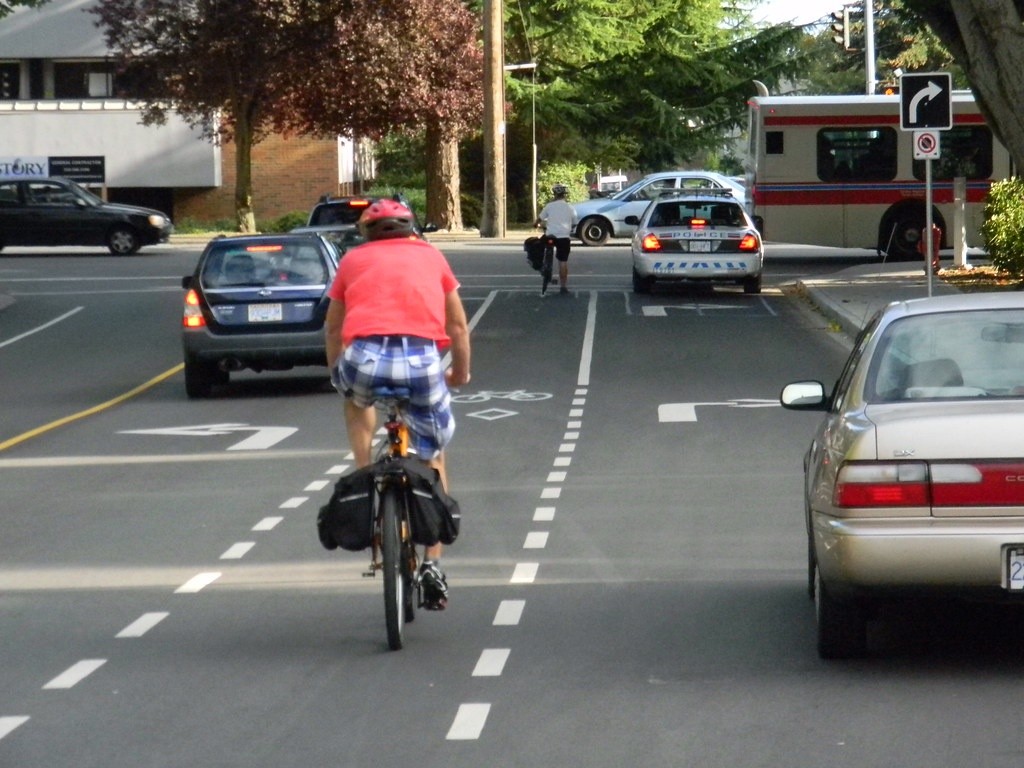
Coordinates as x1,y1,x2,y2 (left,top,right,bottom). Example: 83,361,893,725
915,223,942,273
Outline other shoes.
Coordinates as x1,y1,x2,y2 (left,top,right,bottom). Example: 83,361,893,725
560,286,569,293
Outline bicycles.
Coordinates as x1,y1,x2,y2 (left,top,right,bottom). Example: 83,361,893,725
362,386,447,651
534,224,557,295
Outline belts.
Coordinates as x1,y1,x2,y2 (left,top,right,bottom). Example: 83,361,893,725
368,335,433,347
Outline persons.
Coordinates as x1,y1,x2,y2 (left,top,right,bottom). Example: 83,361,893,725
639,179,676,198
533,185,577,294
323,197,491,606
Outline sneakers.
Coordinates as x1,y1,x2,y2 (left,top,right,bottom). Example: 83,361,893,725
418,560,448,610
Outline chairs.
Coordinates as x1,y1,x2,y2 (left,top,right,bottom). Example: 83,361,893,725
711,206,730,221
898,358,964,391
660,205,681,219
224,255,258,279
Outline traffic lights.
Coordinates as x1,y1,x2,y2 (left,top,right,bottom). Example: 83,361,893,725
830,8,850,49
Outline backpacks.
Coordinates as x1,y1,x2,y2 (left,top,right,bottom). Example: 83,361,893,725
523,236,545,270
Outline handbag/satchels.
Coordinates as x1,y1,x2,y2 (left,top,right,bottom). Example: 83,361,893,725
317,457,461,551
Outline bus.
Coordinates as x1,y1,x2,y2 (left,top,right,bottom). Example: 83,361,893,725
744,77,1018,260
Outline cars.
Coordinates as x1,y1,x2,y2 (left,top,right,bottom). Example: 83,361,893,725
565,171,753,247
273,225,364,293
0,175,173,258
779,291,1024,656
625,185,764,296
180,228,349,398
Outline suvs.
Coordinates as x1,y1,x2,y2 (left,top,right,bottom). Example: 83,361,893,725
305,189,439,259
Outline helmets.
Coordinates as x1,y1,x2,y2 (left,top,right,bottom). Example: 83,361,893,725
357,199,414,240
552,184,566,194
